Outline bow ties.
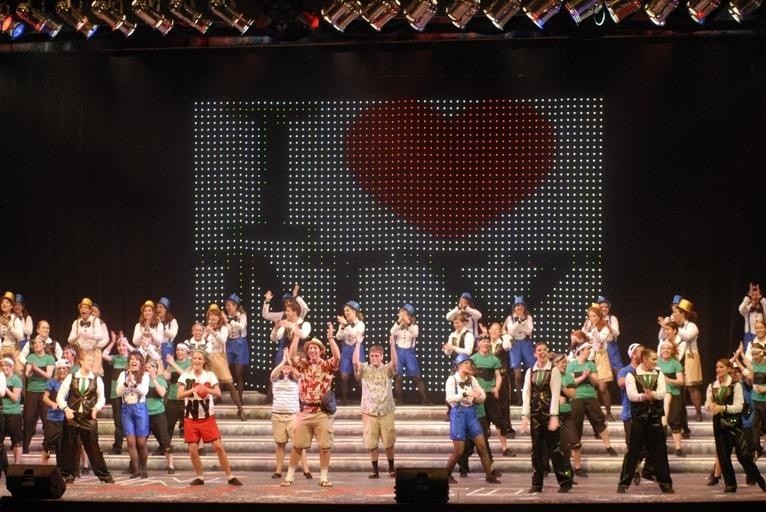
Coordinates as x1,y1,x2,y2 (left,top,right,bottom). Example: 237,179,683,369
514,316,526,323
400,325,410,331
460,379,474,390
80,321,92,328
346,322,355,328
748,305,764,312
227,316,238,322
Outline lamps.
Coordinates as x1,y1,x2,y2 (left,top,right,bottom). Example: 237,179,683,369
0,1,24,40
522,0,562,29
445,0,480,29
643,0,680,26
208,0,255,35
320,1,362,32
168,0,213,34
15,0,61,39
359,0,400,31
564,0,603,27
131,0,174,36
727,0,762,24
686,0,721,25
400,0,437,32
604,0,642,23
91,0,137,38
483,0,521,31
54,0,99,40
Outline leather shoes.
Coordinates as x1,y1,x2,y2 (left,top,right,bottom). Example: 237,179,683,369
260,399,270,406
449,474,457,482
389,470,395,476
339,401,348,406
228,477,244,486
460,471,466,477
272,472,278,480
676,448,684,459
615,486,621,489
515,400,523,407
140,472,148,475
681,431,690,438
318,481,333,487
696,413,701,420
100,475,115,483
281,479,289,484
192,478,208,487
746,478,753,482
529,484,540,490
490,469,501,478
708,476,717,484
607,446,617,456
129,472,136,478
304,472,312,480
237,410,246,420
368,474,378,479
485,475,497,482
214,397,220,405
81,469,87,473
199,448,207,457
558,482,570,490
663,485,672,492
575,469,584,479
112,446,118,454
394,399,402,405
632,472,637,481
153,448,163,453
168,467,176,475
66,477,72,481
389,472,392,475
502,449,517,457
606,412,614,417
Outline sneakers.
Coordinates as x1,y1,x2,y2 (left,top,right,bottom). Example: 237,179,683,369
506,430,515,439
423,402,433,406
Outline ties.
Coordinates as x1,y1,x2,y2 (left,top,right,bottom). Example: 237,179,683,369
719,385,724,400
646,373,650,385
81,378,85,393
538,368,543,385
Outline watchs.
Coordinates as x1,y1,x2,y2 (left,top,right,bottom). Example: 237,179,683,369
263,299,272,304
327,336,337,340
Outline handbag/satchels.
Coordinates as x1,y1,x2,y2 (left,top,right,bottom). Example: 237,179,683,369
323,391,338,414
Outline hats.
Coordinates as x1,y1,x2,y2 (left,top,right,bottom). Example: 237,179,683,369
672,295,679,302
229,294,241,307
78,298,94,310
92,307,100,318
176,340,190,353
12,292,24,304
55,359,72,367
677,298,694,316
159,297,171,311
282,293,295,303
591,302,603,312
455,356,475,366
730,358,740,364
628,343,642,359
206,303,221,317
347,300,361,312
0,291,15,307
575,343,593,353
597,296,612,312
658,340,675,349
303,338,327,356
0,357,16,364
403,304,415,315
514,295,525,307
142,300,157,313
462,292,472,301
33,334,44,341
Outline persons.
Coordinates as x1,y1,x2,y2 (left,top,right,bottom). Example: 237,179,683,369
43,359,72,465
57,349,115,484
117,350,150,480
3,353,23,465
389,304,433,407
459,358,500,478
0,370,10,475
442,313,476,355
268,304,312,351
444,353,499,483
14,293,32,340
468,335,516,457
203,304,245,422
156,296,178,368
565,341,617,457
139,334,161,364
658,299,702,421
213,294,250,405
733,340,752,373
566,330,596,363
581,303,615,422
657,322,690,438
739,283,766,356
0,345,19,376
519,342,572,493
270,348,313,481
746,322,766,363
336,300,365,404
101,329,141,453
704,359,766,493
63,343,88,474
478,320,512,438
23,336,56,452
748,342,766,460
120,359,175,474
133,300,163,350
152,341,205,455
447,293,483,338
177,349,243,487
617,349,675,493
280,321,341,488
92,303,103,319
658,295,686,346
262,284,309,404
19,320,63,367
707,353,754,485
656,343,684,457
617,343,657,485
543,351,588,485
185,322,213,368
0,291,23,347
501,297,536,406
352,331,398,479
597,295,622,374
67,298,109,347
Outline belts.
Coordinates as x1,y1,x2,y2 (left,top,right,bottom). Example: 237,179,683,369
300,401,324,409
274,411,297,416
